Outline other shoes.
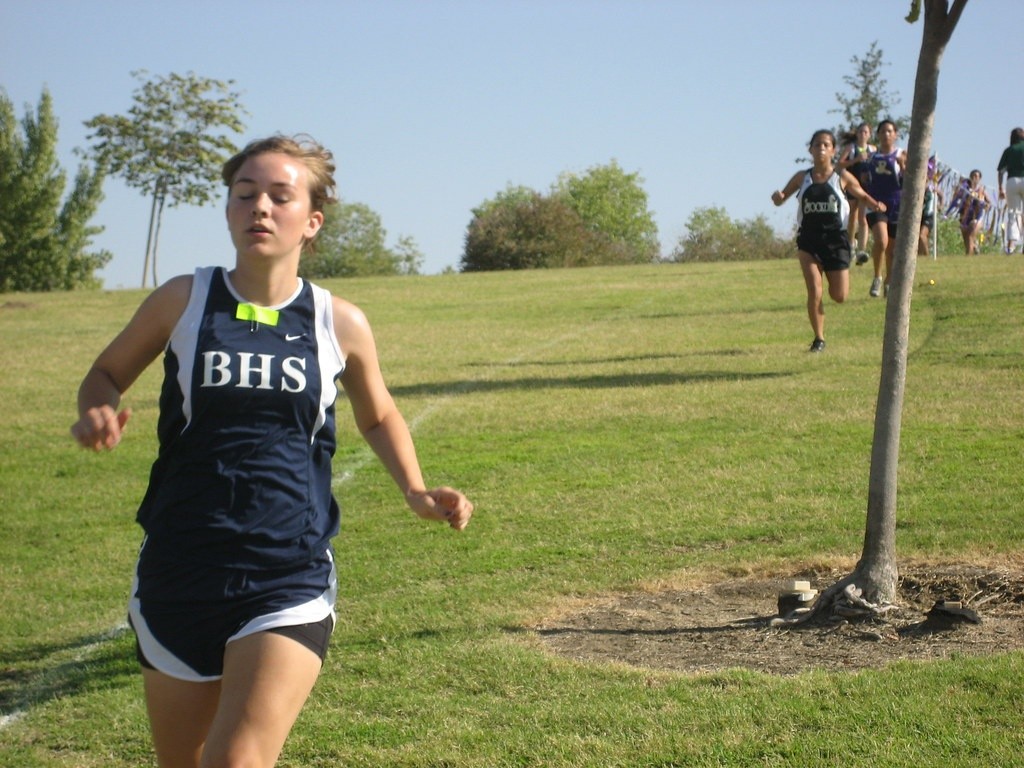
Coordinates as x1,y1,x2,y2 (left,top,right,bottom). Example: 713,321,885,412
974,245,979,255
848,238,858,258
856,251,869,265
1009,247,1015,253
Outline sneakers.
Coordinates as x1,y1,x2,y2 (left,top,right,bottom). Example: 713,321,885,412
885,277,891,296
870,277,883,298
810,337,825,353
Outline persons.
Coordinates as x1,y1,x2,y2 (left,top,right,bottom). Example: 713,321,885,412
70,136,475,768
953,169,990,255
997,128,1024,254
918,178,944,256
861,120,906,296
772,129,887,351
838,124,876,266
